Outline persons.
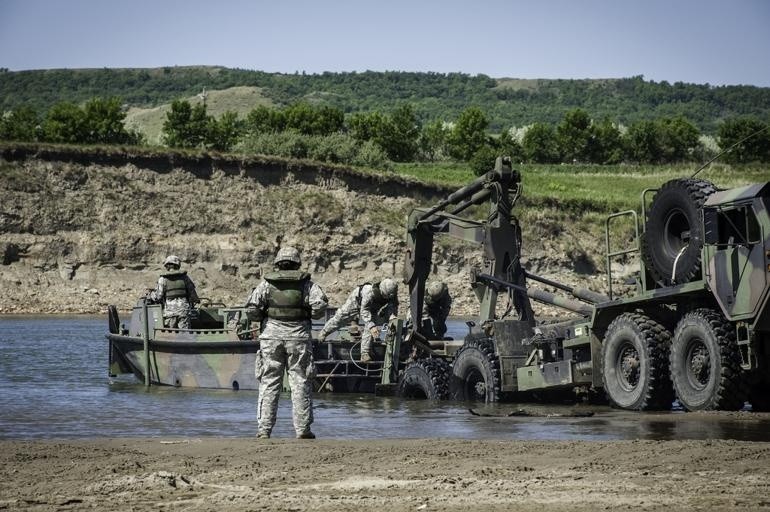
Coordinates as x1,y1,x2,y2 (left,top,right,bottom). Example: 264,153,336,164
244,246,329,438
405,280,452,340
317,278,399,362
150,254,200,334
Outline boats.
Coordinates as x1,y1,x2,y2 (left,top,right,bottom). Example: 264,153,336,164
101,290,465,403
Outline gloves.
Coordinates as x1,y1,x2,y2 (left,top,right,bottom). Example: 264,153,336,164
371,327,378,338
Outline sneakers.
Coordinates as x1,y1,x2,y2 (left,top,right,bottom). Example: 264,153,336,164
318,335,325,342
256,432,270,438
297,431,315,439
361,353,372,361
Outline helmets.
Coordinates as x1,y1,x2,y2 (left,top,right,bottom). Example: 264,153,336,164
379,279,398,299
428,281,444,298
274,247,301,266
164,256,181,268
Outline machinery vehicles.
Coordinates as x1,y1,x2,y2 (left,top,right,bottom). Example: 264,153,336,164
374,153,770,416
